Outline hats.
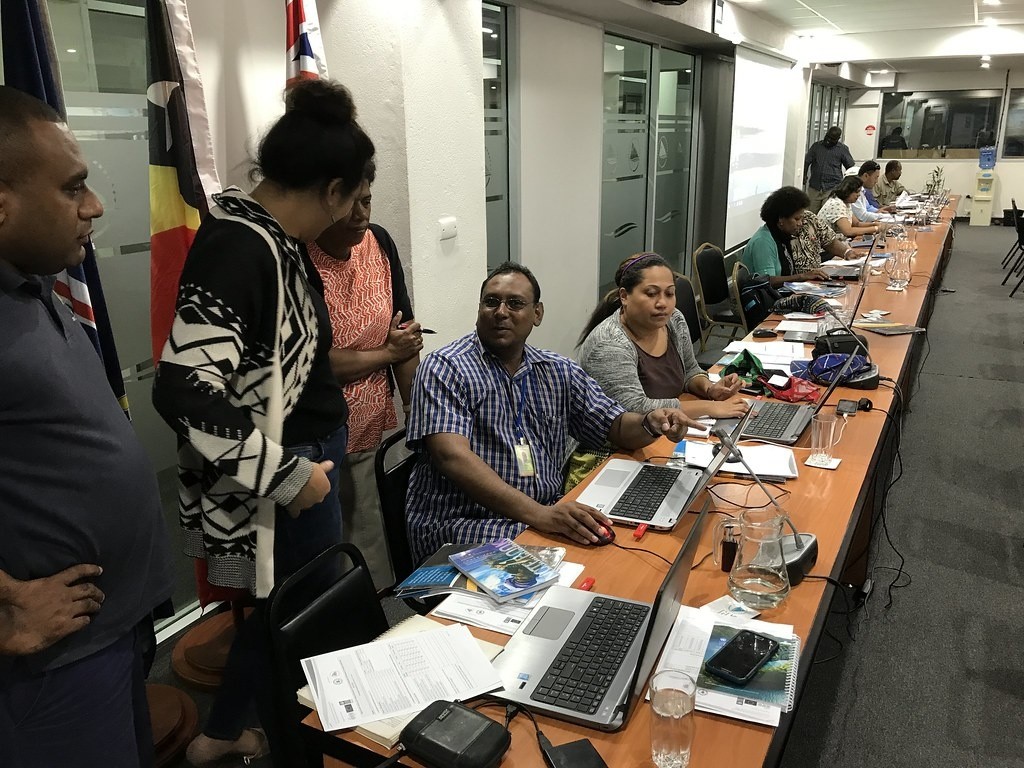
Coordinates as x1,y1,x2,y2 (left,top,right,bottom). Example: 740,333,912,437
844,166,861,176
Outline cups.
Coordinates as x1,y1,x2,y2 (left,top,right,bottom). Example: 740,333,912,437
649,670,698,768
817,311,848,338
858,263,872,287
811,413,838,465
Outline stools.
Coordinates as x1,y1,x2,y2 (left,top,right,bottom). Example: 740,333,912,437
146,684,199,768
171,610,239,695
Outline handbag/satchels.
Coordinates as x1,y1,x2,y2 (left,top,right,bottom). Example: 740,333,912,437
812,328,868,359
740,272,778,329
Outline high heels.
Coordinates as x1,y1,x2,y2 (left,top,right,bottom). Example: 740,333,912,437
186,728,271,768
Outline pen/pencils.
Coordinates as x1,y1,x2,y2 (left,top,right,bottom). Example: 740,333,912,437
864,247,881,248
700,423,714,426
397,323,438,334
717,471,786,484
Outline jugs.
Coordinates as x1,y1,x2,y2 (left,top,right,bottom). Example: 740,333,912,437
712,506,792,610
882,228,918,290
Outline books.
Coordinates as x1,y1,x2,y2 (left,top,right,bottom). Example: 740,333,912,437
695,625,800,712
448,538,559,604
783,282,823,295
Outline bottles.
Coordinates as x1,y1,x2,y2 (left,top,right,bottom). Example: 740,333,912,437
979,146,997,170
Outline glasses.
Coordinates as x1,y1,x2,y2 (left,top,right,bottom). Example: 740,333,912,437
481,297,536,310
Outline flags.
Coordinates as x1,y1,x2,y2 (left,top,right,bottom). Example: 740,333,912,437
285,0,329,97
145,0,231,609
0,0,133,424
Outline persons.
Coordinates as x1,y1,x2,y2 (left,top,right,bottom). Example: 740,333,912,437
790,210,864,275
803,126,856,215
882,127,908,150
0,85,174,768
305,162,423,593
844,160,896,222
741,186,830,289
153,79,376,768
817,176,880,263
404,261,707,571
872,160,910,206
562,251,758,495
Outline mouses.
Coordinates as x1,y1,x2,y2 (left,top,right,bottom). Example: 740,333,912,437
752,328,778,338
712,443,743,463
582,519,616,546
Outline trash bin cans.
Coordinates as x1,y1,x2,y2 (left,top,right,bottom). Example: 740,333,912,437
1004,209,1023,226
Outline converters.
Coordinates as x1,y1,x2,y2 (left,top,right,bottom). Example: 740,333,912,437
544,738,608,768
853,578,874,604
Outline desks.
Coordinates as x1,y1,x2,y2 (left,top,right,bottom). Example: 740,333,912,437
300,194,958,768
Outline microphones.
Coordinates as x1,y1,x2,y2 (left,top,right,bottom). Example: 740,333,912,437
825,305,879,390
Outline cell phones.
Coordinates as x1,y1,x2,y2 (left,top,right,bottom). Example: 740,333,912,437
705,629,779,685
819,281,846,287
836,399,858,416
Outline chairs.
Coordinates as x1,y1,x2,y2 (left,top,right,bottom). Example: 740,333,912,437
266,541,390,768
674,271,727,370
693,242,744,354
374,424,438,614
1001,198,1024,297
732,261,767,335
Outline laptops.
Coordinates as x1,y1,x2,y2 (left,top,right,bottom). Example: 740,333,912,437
849,240,885,249
820,230,881,281
483,497,713,733
709,344,860,447
574,404,753,531
912,183,936,202
782,278,866,344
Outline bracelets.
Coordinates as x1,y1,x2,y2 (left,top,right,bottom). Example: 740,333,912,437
844,249,852,260
873,222,875,226
875,226,878,234
641,410,662,438
706,387,711,400
